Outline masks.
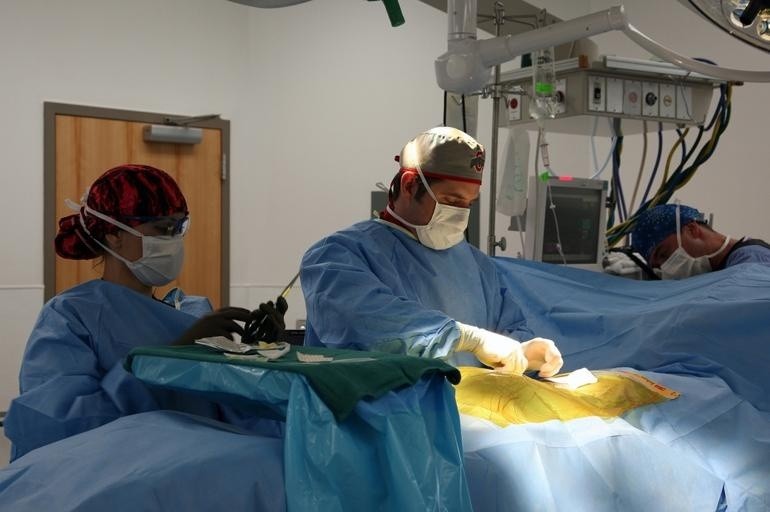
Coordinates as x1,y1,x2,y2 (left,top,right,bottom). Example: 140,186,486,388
659,234,720,281
112,228,186,287
410,195,471,252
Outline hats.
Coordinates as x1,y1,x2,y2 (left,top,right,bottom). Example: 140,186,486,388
631,205,705,259
54,165,190,259
401,126,486,186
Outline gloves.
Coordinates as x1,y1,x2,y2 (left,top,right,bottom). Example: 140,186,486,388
524,337,564,379
457,321,529,375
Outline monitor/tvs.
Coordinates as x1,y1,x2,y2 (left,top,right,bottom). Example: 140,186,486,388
525,175,609,273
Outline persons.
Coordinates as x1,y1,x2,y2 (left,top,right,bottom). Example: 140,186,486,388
3,163,288,464
629,204,770,279
298,125,565,380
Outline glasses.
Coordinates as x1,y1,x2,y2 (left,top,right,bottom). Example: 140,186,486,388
120,210,189,239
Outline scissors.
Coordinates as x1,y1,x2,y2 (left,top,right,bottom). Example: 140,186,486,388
244,271,300,343
